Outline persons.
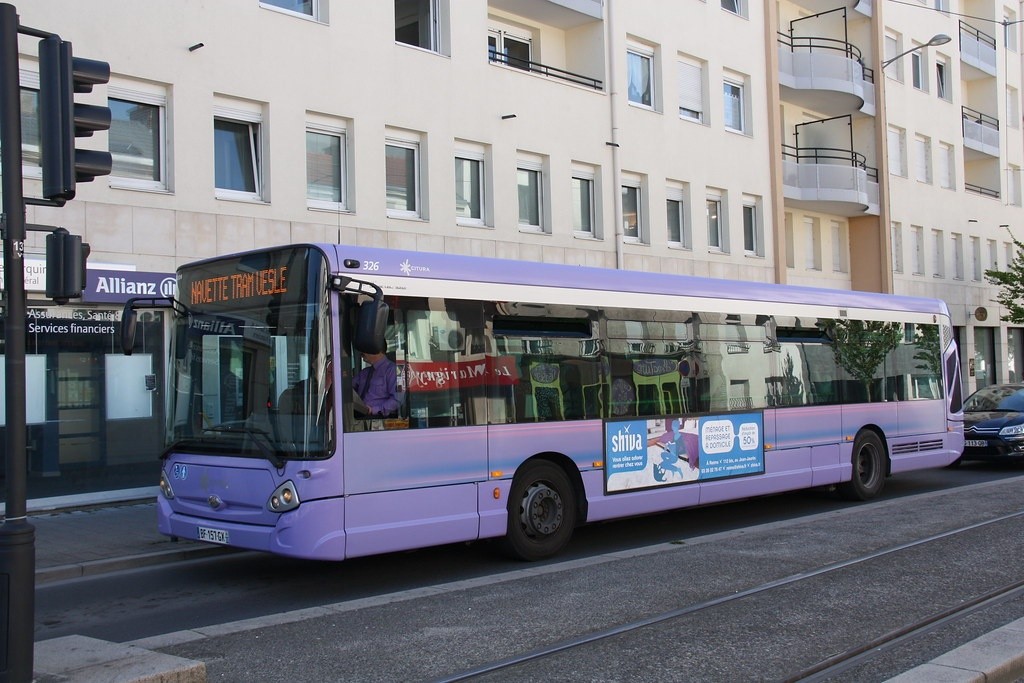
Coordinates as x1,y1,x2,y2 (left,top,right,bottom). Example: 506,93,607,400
811,361,832,403
351,336,405,418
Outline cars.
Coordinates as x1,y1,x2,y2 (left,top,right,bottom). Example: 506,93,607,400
949,382,1024,471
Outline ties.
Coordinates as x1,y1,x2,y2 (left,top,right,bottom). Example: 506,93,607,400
360,365,375,400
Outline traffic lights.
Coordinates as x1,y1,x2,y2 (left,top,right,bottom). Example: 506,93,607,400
37,37,113,200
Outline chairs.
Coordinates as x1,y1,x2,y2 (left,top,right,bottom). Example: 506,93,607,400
387,350,408,418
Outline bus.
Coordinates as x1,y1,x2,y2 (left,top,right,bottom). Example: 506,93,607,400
118,239,965,562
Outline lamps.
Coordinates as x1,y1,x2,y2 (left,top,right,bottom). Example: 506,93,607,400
881,33,952,73
